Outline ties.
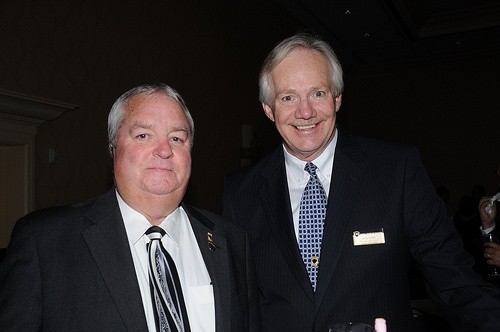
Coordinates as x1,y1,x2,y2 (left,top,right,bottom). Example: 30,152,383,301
145,226,191,332
298,164,328,293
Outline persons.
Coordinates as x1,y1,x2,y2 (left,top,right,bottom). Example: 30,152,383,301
0,81,248,332
477,196,500,267
218,33,500,332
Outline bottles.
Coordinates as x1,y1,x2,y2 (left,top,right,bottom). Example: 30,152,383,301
483,235,500,279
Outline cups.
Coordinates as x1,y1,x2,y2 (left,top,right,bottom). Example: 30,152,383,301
328,322,373,332
